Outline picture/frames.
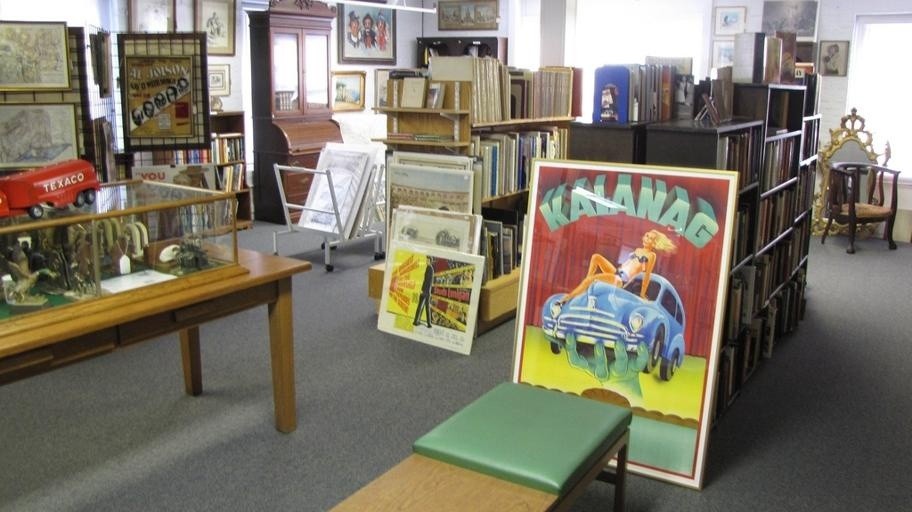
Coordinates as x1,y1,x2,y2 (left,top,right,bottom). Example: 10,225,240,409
510,158,742,490
0,21,72,92
438,0,500,31
0,102,81,172
714,6,747,38
818,40,849,77
194,0,236,57
711,39,735,70
128,0,176,33
116,30,213,152
331,0,411,115
205,63,232,99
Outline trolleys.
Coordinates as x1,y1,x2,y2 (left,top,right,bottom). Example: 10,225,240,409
269,161,385,271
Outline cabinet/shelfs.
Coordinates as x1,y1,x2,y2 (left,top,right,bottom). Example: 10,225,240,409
566,73,822,433
369,80,576,337
124,111,254,244
243,0,344,225
416,37,506,69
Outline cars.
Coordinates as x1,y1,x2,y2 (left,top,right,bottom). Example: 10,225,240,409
540,270,686,382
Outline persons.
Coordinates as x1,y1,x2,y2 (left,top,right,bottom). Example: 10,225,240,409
346,11,388,51
131,78,189,126
825,45,840,74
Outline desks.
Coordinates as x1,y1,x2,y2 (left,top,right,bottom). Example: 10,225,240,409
0,239,312,433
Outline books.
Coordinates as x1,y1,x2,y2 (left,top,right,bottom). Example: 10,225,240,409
172,132,244,234
711,121,819,420
599,56,694,124
276,92,293,111
387,56,575,284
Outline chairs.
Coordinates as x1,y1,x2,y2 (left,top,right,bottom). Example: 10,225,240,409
821,161,901,253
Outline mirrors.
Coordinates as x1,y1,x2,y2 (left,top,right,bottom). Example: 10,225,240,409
810,108,891,236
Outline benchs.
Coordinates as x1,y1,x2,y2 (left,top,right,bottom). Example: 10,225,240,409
327,380,632,512
